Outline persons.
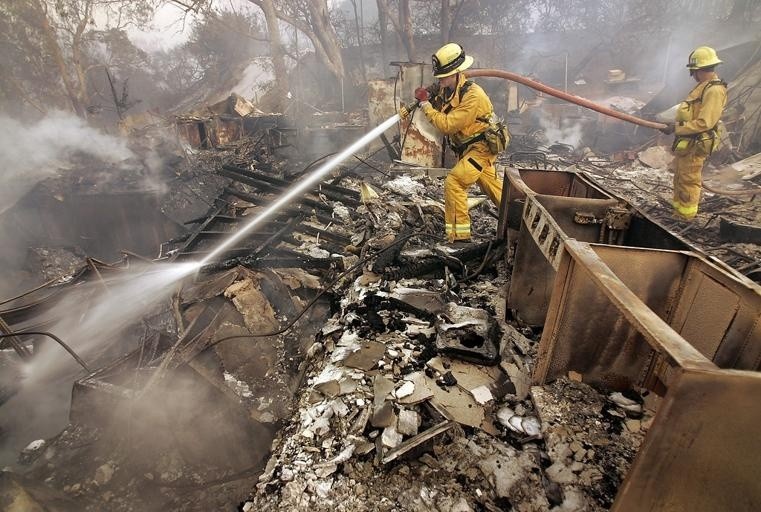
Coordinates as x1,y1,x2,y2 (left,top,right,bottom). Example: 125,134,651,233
661,46,729,221
414,43,511,244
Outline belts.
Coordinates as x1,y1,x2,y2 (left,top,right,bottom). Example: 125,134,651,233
458,133,484,153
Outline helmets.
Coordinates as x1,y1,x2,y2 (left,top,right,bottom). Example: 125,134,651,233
687,46,724,69
432,43,474,79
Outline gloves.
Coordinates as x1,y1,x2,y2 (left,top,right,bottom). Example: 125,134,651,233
659,122,674,134
415,88,427,102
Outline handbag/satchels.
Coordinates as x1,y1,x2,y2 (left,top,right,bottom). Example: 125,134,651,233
485,122,511,154
694,120,729,155
